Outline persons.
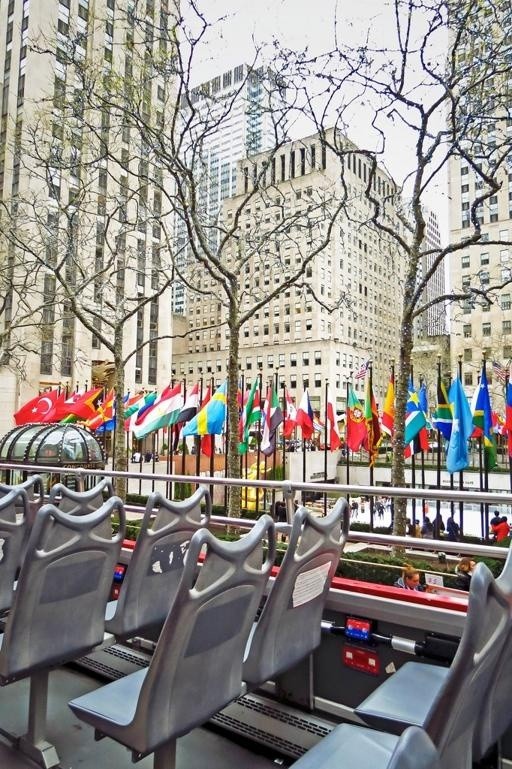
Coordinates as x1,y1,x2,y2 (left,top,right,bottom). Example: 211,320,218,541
267,494,511,542
451,558,477,592
391,562,425,591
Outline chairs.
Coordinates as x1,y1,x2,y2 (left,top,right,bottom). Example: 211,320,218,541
0,473,212,769
62,497,350,769
286,538,512,769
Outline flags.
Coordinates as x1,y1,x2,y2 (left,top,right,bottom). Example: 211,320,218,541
11,361,511,473
